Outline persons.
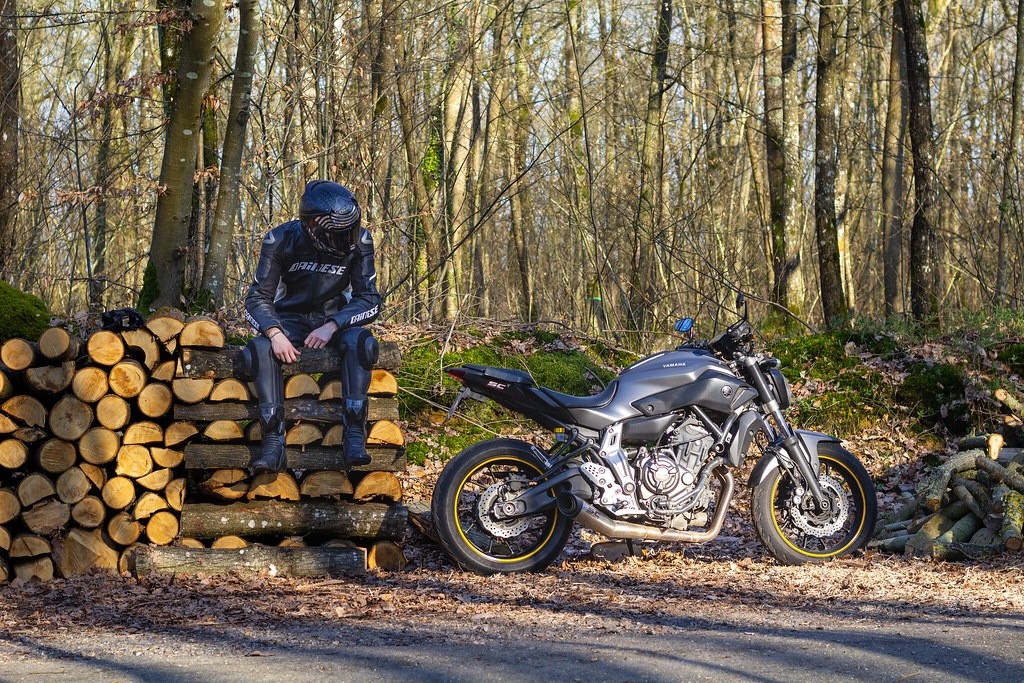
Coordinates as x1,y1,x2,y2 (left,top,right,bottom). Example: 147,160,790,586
240,179,384,474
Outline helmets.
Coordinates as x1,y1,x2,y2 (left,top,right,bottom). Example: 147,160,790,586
299,180,362,260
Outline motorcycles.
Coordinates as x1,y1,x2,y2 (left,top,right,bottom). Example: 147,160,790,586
430,292,878,577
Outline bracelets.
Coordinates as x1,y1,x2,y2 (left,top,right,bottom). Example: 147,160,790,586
269,331,282,340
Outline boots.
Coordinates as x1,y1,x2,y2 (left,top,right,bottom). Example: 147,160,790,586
342,394,371,466
253,404,288,474
